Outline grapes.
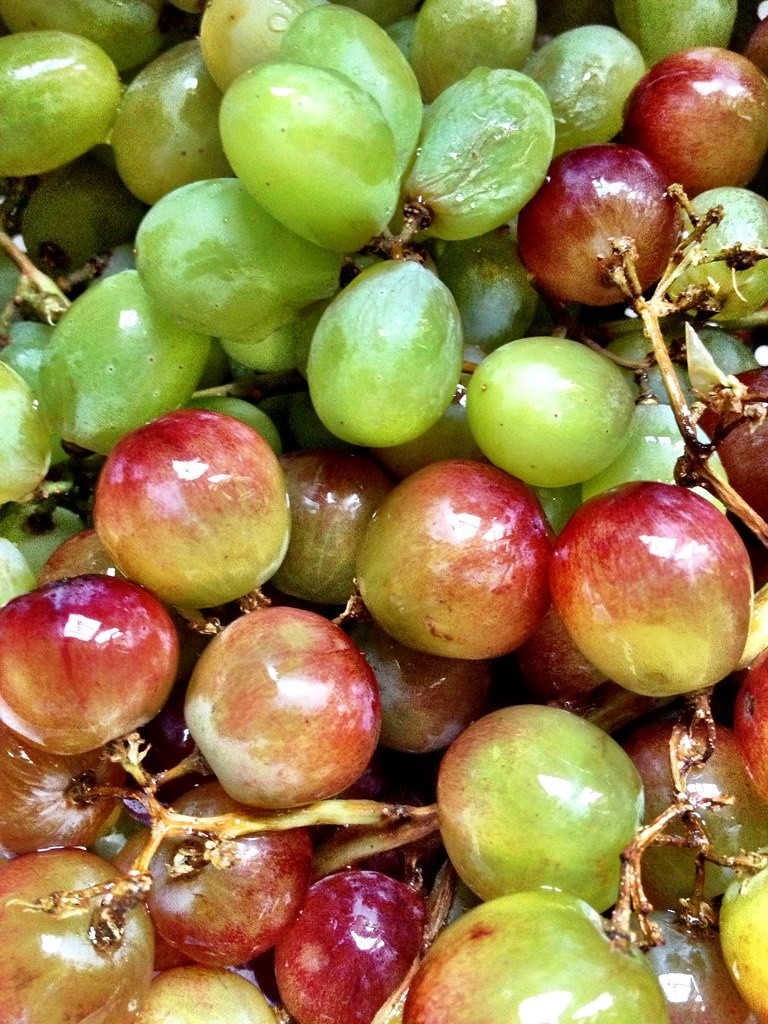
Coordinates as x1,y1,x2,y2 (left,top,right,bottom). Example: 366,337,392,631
0,0,768,1024
